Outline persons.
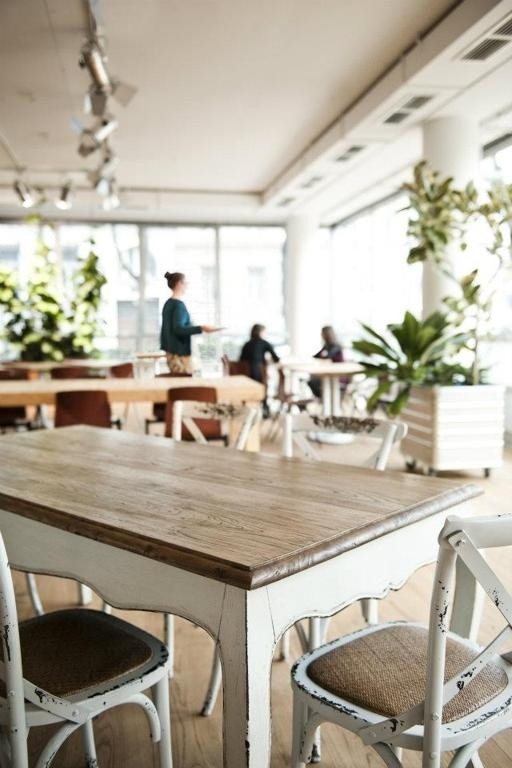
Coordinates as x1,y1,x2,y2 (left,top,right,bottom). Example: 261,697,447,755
308,325,352,401
159,271,216,375
239,323,279,417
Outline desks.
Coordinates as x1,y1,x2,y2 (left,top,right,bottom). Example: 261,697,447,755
2,421,488,761
1,349,385,450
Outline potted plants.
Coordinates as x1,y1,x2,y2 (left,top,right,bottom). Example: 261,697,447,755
352,161,510,478
0,219,111,365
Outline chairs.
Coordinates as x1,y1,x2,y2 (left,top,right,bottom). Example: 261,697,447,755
1,525,175,763
286,513,512,763
1,349,385,450
200,408,407,719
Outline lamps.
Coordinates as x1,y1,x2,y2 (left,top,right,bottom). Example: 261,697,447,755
1,2,139,211
